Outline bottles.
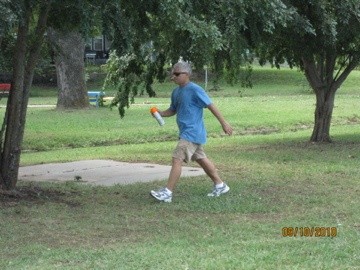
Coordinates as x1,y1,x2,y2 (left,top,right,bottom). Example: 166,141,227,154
151,107,165,126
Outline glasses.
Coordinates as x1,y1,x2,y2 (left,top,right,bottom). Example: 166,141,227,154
173,72,188,77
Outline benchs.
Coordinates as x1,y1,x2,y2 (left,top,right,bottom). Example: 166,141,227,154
87,91,106,108
0,84,11,99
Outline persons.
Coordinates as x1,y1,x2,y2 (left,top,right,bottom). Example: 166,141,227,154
150,62,233,203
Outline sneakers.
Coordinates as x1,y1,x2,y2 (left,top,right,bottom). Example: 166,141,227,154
207,182,231,197
149,188,173,204
